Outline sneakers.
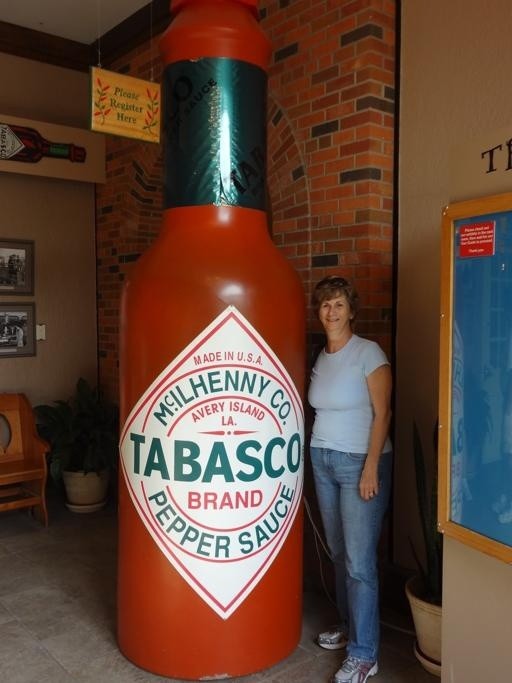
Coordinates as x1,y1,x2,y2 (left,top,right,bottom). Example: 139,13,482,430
333,654,378,683
317,625,350,650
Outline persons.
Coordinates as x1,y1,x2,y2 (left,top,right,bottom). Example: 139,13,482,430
15,323,24,350
307,274,395,682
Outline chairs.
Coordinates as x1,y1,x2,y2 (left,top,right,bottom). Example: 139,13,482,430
0,392,51,529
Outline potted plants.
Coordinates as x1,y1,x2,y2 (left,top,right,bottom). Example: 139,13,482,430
405,418,444,678
32,376,120,515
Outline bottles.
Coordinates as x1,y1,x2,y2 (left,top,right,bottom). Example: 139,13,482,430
116,0,309,681
0,123,86,164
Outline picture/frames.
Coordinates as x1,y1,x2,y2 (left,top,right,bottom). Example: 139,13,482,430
434,190,512,567
0,238,37,358
